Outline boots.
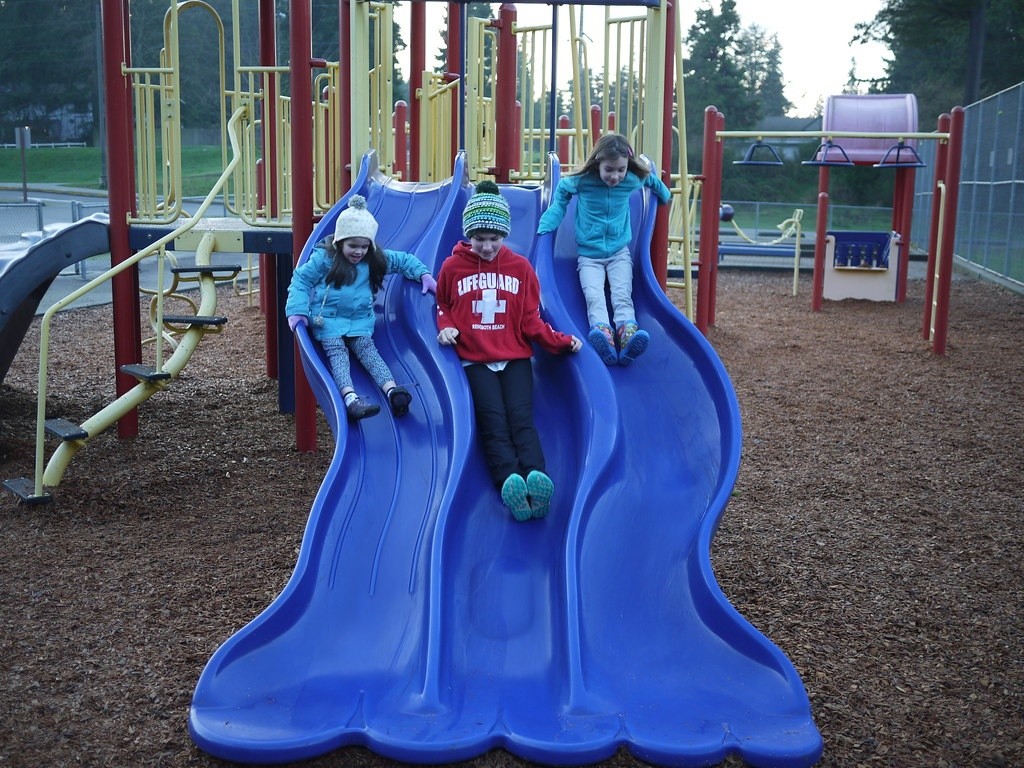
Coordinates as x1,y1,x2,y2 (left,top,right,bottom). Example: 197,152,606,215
619,320,650,368
588,323,618,366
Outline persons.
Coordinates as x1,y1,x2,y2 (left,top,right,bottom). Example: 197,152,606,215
536,136,672,366
285,194,438,424
434,180,583,521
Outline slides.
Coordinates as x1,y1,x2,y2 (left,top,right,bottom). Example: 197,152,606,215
186,150,827,768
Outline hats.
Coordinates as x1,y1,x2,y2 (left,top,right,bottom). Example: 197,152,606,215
333,194,378,252
462,180,511,239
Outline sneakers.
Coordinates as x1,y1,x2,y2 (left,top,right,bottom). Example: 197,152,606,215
390,386,413,418
346,398,380,420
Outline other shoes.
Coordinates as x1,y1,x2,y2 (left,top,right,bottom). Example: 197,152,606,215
501,473,533,521
527,470,554,519
